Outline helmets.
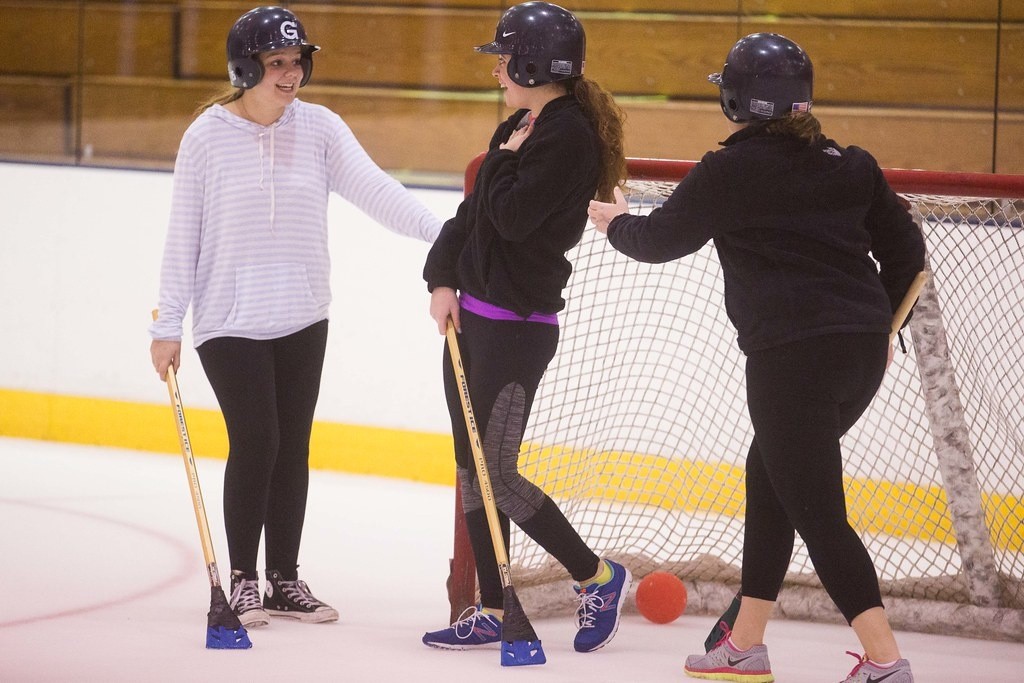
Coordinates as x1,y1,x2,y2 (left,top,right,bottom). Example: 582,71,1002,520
475,0,587,88
226,6,321,88
705,32,814,123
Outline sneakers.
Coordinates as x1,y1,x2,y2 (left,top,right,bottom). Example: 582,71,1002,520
573,559,632,652
686,619,774,683
264,571,338,624
228,569,269,628
841,650,915,683
422,603,503,651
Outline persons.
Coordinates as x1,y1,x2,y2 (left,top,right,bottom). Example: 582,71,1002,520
151,5,443,628
589,32,926,683
423,2,633,653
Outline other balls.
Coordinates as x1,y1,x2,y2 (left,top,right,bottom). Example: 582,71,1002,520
634,572,684,621
